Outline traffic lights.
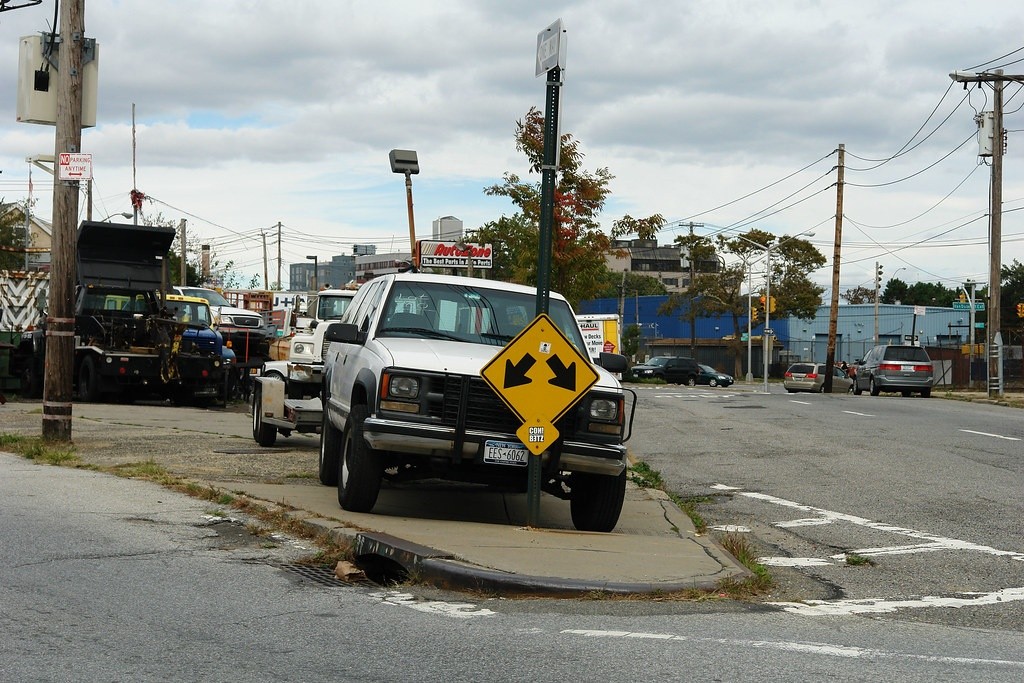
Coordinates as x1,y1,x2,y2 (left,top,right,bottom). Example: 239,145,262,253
960,292,966,304
759,296,767,313
769,296,778,313
1017,303,1024,318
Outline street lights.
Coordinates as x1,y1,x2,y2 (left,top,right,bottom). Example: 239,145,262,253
893,268,907,278
727,231,817,397
721,249,780,380
306,255,318,291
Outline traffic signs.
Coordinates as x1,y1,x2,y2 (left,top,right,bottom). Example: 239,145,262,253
905,334,919,341
57,153,93,181
534,19,562,77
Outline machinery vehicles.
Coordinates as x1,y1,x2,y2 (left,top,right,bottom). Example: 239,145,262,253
0,220,242,410
250,286,418,446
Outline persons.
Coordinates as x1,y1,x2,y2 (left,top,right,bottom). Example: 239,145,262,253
841,361,850,376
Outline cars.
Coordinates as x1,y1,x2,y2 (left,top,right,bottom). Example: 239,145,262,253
854,345,934,398
698,363,734,387
784,362,855,394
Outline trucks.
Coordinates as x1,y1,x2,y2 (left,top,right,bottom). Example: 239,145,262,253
566,314,623,384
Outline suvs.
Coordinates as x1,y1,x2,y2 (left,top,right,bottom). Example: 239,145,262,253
320,273,638,533
169,285,266,353
630,356,701,387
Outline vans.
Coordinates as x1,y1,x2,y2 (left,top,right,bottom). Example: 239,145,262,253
121,295,214,331
